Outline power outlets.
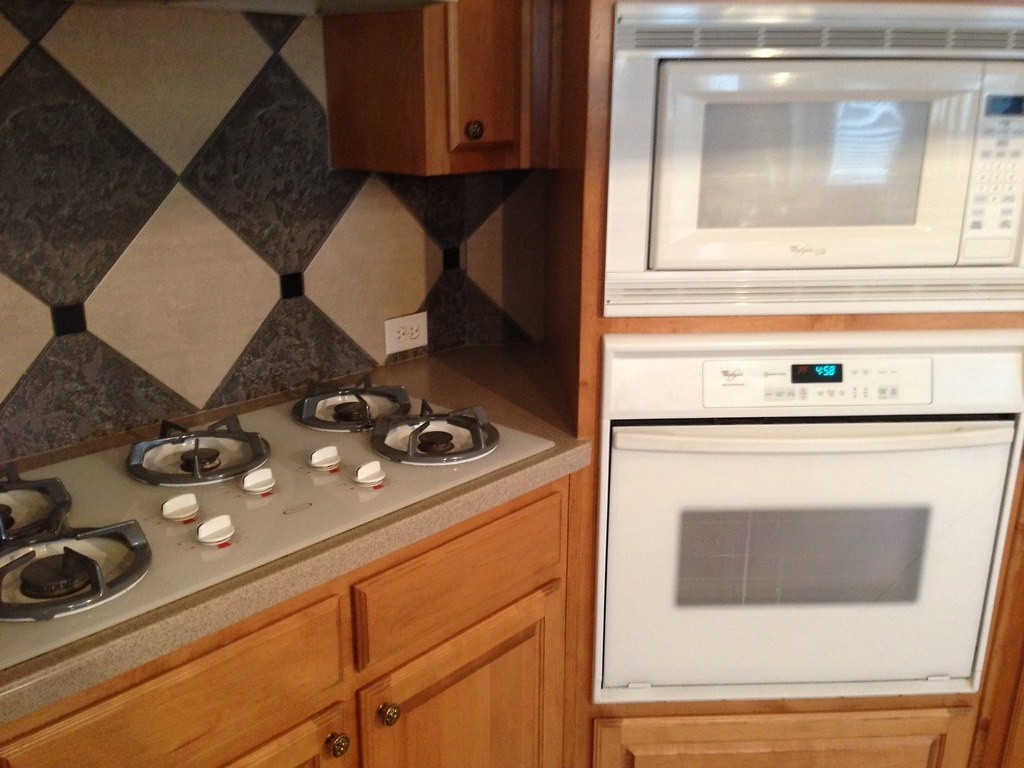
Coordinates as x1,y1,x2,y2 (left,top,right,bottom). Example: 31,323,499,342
384,311,428,355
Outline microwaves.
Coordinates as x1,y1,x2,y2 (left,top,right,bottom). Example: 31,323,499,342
602,1,1024,318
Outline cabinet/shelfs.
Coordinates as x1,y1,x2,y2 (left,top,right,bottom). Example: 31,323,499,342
319,1,563,179
0,478,571,768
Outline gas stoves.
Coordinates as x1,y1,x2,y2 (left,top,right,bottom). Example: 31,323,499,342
0,358,594,734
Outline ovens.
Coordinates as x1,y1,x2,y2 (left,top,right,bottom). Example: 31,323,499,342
596,330,1023,704
